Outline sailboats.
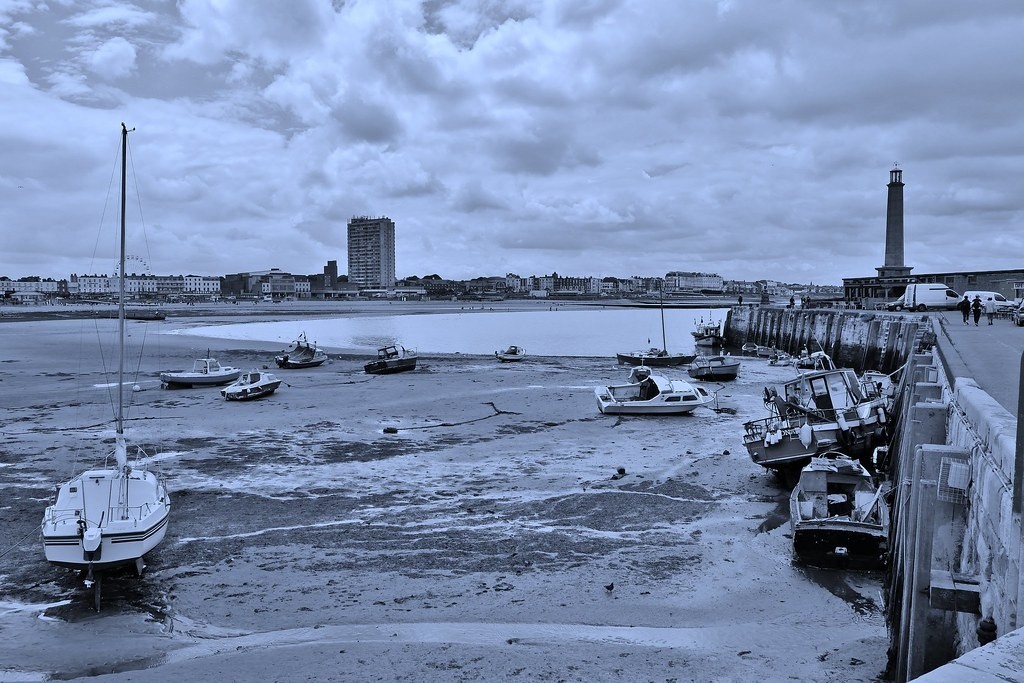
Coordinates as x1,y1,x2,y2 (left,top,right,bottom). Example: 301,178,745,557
616,280,698,367
39,121,172,576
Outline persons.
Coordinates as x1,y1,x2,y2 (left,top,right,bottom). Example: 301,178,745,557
789,295,811,310
187,300,293,306
556,308,557,311
462,306,510,311
960,294,997,326
738,294,743,306
550,307,551,311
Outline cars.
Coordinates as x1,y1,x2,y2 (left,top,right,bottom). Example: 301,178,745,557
1009,296,1024,327
885,293,904,312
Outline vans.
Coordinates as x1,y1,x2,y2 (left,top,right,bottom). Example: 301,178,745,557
962,290,1014,314
904,282,964,312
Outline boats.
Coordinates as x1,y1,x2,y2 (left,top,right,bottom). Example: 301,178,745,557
221,366,282,402
495,345,528,362
687,353,741,381
274,330,328,369
594,356,717,415
788,451,892,570
740,326,908,483
364,344,419,375
160,347,243,389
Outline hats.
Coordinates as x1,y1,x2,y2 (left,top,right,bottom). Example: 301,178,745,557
976,295,980,297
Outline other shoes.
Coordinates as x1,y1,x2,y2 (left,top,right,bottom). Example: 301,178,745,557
966,321,970,325
964,323,965,326
989,322,990,325
975,323,978,326
991,323,993,325
